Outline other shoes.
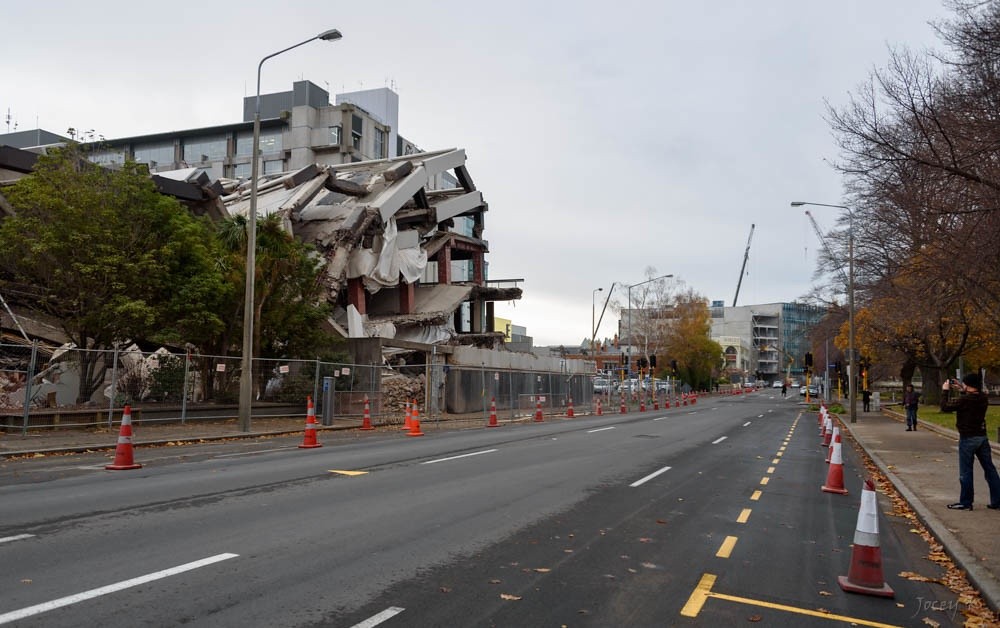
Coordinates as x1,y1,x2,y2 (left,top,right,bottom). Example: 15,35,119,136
864,410,869,412
905,428,912,431
914,427,916,431
946,502,973,511
986,504,1000,510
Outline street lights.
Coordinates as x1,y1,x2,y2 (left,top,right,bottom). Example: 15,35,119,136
592,288,603,361
791,201,857,423
238,28,343,430
628,275,673,404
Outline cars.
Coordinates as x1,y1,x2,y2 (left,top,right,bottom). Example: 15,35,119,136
594,377,672,394
744,380,818,398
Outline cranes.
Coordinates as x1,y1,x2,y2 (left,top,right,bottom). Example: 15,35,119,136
732,211,849,307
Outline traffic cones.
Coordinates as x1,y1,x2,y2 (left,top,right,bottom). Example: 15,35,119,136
837,478,894,597
487,396,500,427
533,397,545,422
820,415,832,446
105,406,143,469
817,400,829,437
820,435,848,495
825,418,845,465
401,396,424,436
360,400,375,430
566,395,696,418
297,402,321,448
303,395,319,424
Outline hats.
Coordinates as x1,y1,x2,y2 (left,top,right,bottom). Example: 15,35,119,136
962,373,982,390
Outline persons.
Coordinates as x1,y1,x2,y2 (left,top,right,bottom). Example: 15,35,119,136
902,384,918,431
861,388,872,412
781,384,787,397
941,373,1000,510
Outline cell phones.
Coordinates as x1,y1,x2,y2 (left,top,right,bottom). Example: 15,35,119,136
949,379,954,390
